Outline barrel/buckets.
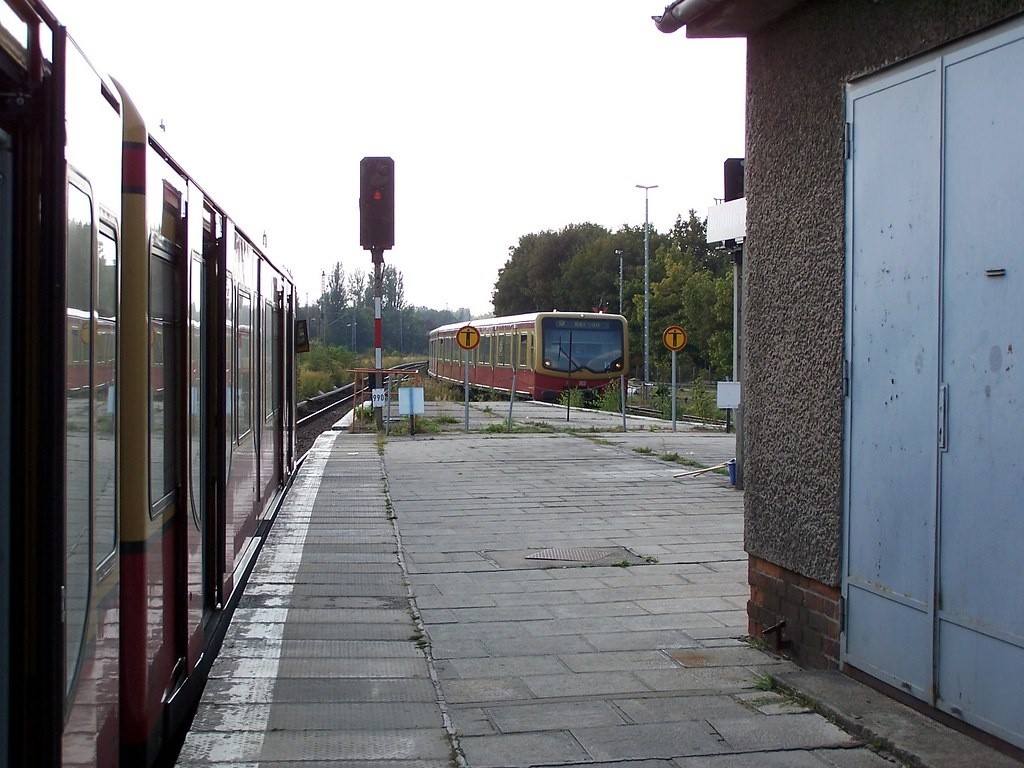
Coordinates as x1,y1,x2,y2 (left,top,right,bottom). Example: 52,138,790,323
727,460,736,485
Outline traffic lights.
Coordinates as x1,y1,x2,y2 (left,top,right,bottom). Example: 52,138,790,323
359,157,395,252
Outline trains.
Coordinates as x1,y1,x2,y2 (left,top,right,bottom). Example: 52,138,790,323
427,308,630,407
0,0,311,768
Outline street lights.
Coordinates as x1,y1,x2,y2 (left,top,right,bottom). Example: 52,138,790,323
636,185,659,382
614,249,624,317
